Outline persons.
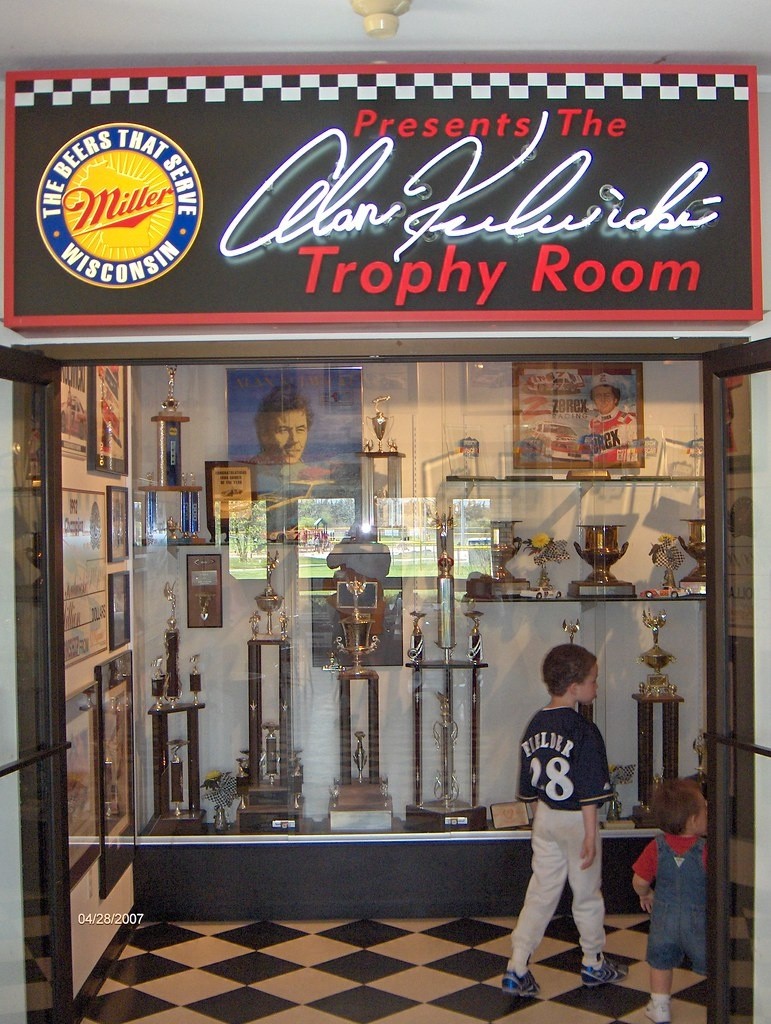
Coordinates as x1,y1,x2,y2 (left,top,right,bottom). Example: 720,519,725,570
588,373,637,459
299,532,332,552
323,516,393,651
632,778,708,1023
499,643,630,998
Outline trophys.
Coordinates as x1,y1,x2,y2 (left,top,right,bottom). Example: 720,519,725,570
148,363,707,829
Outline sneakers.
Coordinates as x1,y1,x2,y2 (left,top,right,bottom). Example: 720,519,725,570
580,959,628,985
502,968,542,995
644,999,671,1023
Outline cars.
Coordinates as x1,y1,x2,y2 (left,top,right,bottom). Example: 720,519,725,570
525,421,593,460
518,584,565,600
269,524,323,542
639,586,692,599
